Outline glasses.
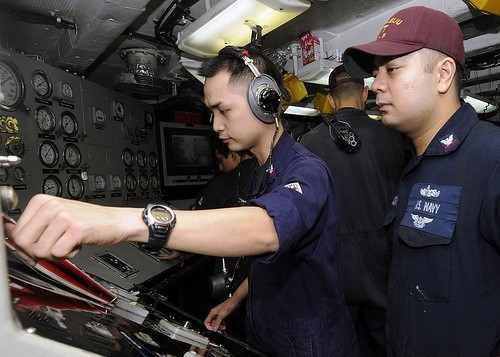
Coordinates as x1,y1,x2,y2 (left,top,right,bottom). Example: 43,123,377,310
215,156,224,165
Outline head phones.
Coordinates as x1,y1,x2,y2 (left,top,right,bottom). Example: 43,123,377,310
321,112,361,154
219,46,283,124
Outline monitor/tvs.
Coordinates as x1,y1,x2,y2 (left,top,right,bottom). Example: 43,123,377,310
160,122,221,186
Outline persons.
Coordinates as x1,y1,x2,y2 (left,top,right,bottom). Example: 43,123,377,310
160,140,259,270
298,63,415,357
339,6,499,357
13,46,358,357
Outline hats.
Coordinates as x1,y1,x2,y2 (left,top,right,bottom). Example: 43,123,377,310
329,64,364,91
342,6,464,80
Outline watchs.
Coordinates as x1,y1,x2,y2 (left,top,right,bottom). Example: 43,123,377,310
138,202,176,254
